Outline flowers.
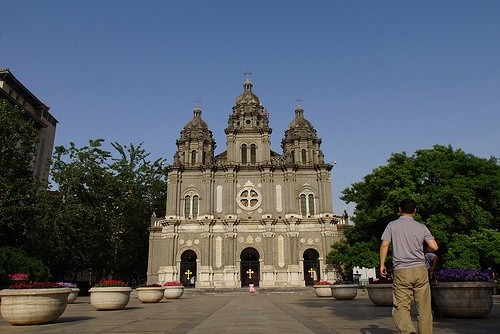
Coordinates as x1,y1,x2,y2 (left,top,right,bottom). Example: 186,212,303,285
369,277,393,284
8,274,27,279
430,268,490,282
333,280,353,285
93,280,128,287
164,282,183,286
317,281,332,285
9,282,63,289
58,283,76,287
137,284,162,287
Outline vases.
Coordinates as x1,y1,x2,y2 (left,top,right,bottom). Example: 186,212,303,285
313,285,332,297
136,288,166,303
330,284,359,301
67,288,80,304
367,284,393,307
430,282,495,318
0,289,73,325
88,287,132,310
165,286,184,299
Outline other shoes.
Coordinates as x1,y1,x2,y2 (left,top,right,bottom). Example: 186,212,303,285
434,307,440,320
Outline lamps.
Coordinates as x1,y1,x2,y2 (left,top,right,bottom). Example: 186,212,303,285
162,161,170,166
331,161,337,165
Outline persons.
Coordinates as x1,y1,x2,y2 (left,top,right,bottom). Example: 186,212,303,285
379,199,439,334
